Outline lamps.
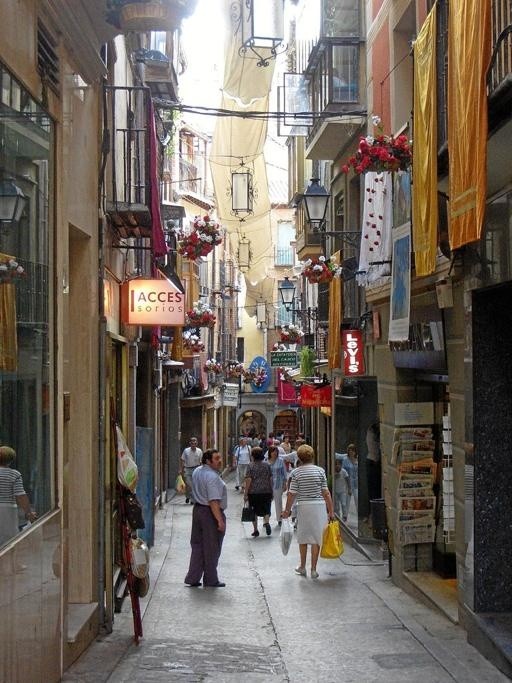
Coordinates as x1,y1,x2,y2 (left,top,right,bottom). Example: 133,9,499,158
236,0,289,67
278,276,320,321
230,154,254,223
256,294,270,330
1,175,30,234
299,177,361,250
236,230,252,273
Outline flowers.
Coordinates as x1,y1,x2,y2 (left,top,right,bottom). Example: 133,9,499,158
300,255,343,283
177,214,224,259
1,255,27,284
186,300,305,386
340,132,412,175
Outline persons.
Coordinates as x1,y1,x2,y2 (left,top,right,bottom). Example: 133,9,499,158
0,446,38,551
179,416,308,536
342,444,359,518
280,444,337,578
334,458,352,521
183,448,229,587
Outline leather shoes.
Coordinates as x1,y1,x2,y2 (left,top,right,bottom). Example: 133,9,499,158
295,567,307,575
266,524,271,535
203,581,225,588
186,498,193,504
311,570,319,578
251,530,259,536
184,579,201,587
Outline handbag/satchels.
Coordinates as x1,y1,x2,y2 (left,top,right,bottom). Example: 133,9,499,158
116,426,139,494
129,535,150,598
122,486,145,533
241,500,257,523
320,517,344,559
